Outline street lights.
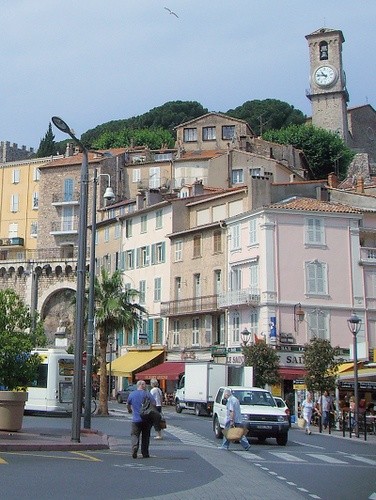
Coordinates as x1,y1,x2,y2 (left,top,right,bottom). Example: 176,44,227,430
83,169,115,429
241,328,252,366
51,115,106,442
346,312,363,439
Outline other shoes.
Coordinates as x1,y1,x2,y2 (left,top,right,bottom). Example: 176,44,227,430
244,445,251,451
143,454,149,458
307,431,312,435
219,446,230,450
132,448,138,459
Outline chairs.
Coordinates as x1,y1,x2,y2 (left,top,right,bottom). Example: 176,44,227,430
244,397,251,402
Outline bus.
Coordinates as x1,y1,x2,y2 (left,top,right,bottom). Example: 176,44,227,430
20,347,75,412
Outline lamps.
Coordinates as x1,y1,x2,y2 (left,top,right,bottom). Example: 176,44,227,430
293,303,305,331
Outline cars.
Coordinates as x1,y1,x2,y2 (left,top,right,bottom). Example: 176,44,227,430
242,395,290,416
116,385,152,403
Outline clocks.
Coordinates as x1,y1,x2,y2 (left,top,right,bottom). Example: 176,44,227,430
312,64,338,89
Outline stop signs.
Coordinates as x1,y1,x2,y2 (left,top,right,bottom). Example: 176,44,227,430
82,351,87,365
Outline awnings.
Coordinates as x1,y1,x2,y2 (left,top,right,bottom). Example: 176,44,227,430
136,362,185,381
272,368,309,381
97,350,164,378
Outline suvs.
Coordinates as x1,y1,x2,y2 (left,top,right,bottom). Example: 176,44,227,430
212,386,289,446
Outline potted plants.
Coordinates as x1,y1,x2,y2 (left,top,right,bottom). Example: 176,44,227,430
0,288,48,430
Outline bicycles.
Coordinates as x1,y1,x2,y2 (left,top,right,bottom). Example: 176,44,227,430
83,399,97,414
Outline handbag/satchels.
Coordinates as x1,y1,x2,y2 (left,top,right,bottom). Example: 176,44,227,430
158,411,167,430
222,424,244,442
297,415,307,428
140,391,163,422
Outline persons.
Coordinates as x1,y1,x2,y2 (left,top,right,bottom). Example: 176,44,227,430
218,389,251,450
127,380,157,458
148,380,163,440
302,390,358,434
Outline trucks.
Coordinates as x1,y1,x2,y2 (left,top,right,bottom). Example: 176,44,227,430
175,362,254,416
